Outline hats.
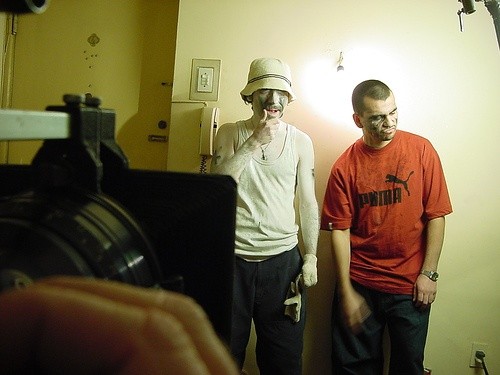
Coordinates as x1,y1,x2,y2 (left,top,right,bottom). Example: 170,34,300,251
240,58,294,104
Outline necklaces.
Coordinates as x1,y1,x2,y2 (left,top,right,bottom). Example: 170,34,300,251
250,119,270,160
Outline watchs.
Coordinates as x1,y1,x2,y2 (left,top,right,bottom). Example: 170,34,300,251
419,269,439,282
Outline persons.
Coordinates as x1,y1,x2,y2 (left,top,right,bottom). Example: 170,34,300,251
320,79,453,375
210,57,320,375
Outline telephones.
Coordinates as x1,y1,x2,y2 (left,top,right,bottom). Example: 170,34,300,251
199,107,219,155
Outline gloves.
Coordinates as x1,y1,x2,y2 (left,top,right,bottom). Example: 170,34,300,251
283,274,303,323
302,254,318,288
